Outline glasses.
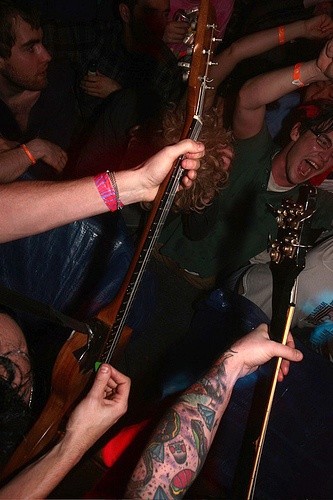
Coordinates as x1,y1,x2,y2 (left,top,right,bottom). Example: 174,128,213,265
308,126,333,151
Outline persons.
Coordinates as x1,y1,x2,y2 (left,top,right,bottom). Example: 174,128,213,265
0,0,333,500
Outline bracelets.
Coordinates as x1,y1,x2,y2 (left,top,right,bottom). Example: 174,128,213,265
278,25,285,44
93,171,124,213
22,143,35,164
292,62,305,88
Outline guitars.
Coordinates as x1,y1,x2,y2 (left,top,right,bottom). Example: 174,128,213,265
223,183,320,500
1,0,224,485
